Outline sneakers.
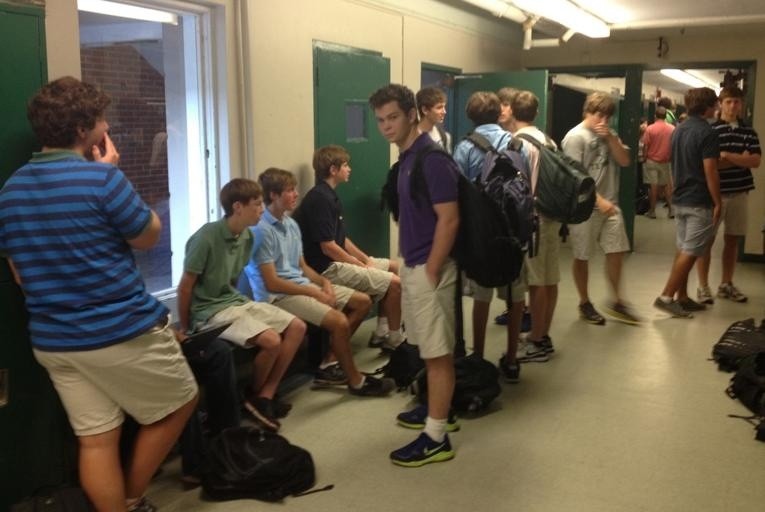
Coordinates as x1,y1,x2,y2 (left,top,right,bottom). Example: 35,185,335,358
245,397,280,429
579,301,641,324
314,363,393,396
495,306,554,383
655,281,747,318
390,408,460,467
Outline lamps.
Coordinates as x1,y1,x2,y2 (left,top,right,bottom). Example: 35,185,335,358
515,0,613,39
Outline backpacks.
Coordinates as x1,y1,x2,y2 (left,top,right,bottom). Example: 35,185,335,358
197,427,314,501
370,338,500,412
408,133,595,287
707,319,765,441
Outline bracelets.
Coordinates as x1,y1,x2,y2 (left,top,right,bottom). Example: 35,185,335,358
720,151,727,162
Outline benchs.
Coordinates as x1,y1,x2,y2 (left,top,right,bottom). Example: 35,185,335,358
167,321,330,418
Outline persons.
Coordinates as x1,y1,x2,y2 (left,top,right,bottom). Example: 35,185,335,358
366,83,463,468
491,88,537,338
513,92,561,362
561,94,643,328
177,181,308,429
241,166,396,398
1,77,200,512
696,86,763,304
639,84,728,219
415,89,453,157
459,89,531,381
290,145,406,352
651,88,722,317
